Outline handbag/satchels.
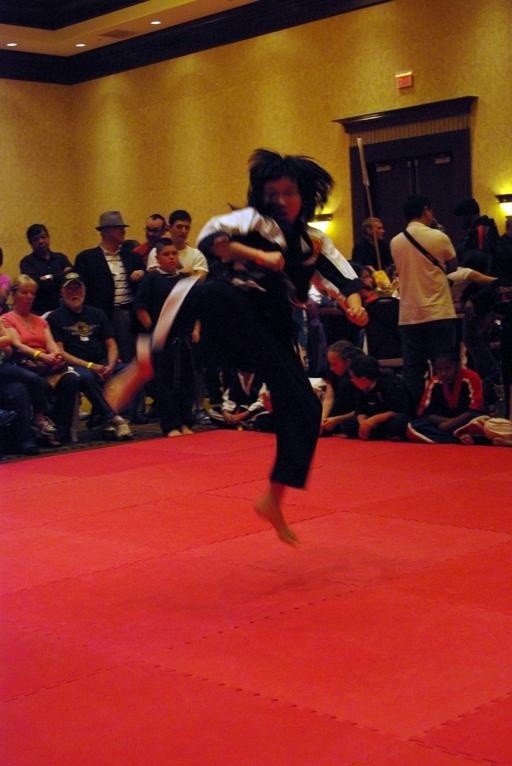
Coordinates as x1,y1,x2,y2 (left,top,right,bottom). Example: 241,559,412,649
20,350,69,375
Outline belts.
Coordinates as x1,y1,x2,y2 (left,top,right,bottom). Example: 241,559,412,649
113,303,131,311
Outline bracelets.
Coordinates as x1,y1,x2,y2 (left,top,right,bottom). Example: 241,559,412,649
33,351,42,358
87,362,93,369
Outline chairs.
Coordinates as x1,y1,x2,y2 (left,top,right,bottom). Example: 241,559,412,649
6,309,123,444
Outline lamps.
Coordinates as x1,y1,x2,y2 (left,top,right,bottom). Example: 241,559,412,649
307,214,333,233
495,194,511,233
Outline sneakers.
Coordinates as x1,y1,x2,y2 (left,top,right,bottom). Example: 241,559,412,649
20,442,38,455
87,410,160,441
0,410,16,425
30,418,61,446
193,409,210,424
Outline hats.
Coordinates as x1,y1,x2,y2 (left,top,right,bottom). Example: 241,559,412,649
95,211,129,231
63,268,84,289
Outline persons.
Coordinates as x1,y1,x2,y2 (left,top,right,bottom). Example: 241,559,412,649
390,198,472,446
408,199,511,446
196,370,273,432
107,148,368,545
1,212,209,445
295,216,409,443
